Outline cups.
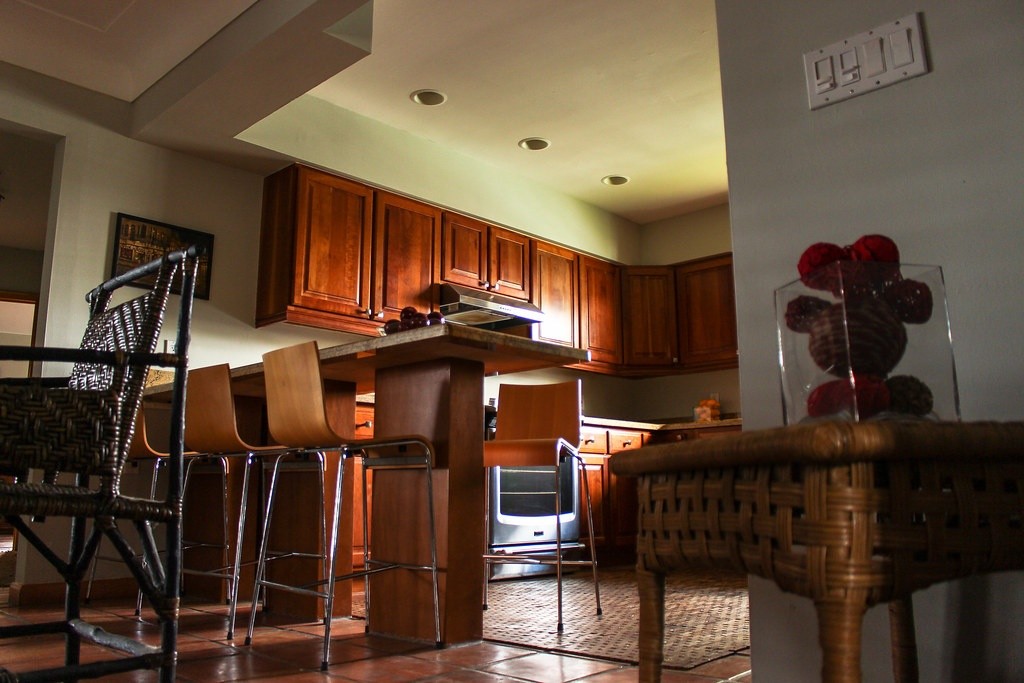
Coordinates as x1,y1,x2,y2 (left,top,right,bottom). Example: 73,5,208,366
774,259,959,427
489,398,497,432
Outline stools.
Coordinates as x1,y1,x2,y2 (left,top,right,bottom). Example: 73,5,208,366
113,340,604,650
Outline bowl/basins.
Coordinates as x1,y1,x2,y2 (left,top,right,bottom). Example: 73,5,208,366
376,326,386,336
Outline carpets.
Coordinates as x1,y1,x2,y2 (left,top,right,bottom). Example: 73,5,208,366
352,566,752,670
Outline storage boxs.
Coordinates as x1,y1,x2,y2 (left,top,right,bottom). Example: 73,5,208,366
773,261,962,425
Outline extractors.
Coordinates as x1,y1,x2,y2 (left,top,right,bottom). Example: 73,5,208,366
440,283,544,331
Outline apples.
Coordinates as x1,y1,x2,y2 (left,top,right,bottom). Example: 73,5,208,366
384,306,445,334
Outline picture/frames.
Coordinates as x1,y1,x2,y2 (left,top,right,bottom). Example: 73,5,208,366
108,212,216,300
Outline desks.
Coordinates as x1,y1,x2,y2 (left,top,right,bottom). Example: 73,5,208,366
132,322,592,650
609,415,1024,683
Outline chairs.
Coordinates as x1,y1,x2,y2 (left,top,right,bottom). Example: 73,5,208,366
0,244,209,682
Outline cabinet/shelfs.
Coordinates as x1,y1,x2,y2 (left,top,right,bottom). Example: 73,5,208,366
254,165,443,337
530,240,625,375
627,250,738,379
577,427,657,555
443,210,531,301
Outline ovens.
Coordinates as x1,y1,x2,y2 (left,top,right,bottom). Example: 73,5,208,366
488,458,582,579
